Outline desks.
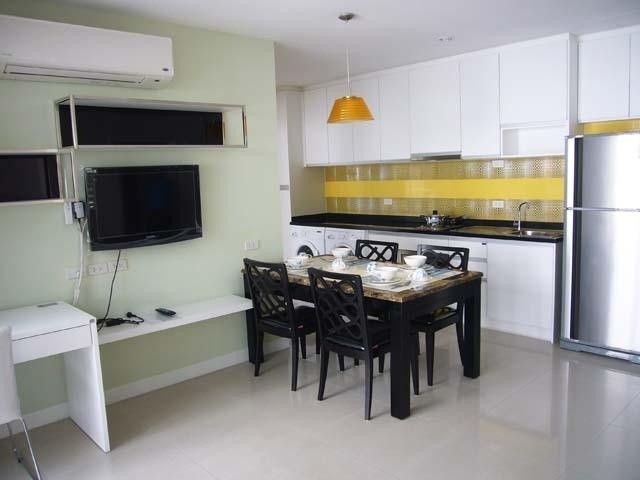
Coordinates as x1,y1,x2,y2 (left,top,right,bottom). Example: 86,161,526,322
0,301,110,455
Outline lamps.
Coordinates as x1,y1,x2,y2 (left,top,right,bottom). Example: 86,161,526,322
325,11,375,127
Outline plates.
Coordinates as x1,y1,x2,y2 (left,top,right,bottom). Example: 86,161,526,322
285,263,314,271
320,255,359,262
400,264,433,271
361,275,406,287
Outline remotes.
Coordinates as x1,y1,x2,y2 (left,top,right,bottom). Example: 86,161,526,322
155,308,176,316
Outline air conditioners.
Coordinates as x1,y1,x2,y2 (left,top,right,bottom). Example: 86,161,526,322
0,13,176,91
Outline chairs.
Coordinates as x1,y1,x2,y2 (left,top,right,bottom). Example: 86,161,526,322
307,267,421,420
242,258,346,393
378,244,470,386
315,239,400,366
0,324,40,480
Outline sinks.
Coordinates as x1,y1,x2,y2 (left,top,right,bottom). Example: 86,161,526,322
508,231,564,238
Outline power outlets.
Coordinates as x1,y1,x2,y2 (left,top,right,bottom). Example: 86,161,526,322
66,266,88,281
88,262,108,275
108,259,128,274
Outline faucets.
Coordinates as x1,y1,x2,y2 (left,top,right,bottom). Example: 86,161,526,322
512,201,530,230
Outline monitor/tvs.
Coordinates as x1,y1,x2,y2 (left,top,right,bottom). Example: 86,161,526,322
83,164,203,252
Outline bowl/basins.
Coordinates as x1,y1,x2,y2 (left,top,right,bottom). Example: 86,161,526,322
403,255,427,269
288,256,309,267
373,267,401,281
332,247,351,259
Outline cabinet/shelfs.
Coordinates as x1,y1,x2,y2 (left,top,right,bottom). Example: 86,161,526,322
485,239,562,344
500,31,580,160
461,45,501,161
369,234,448,263
0,148,80,206
448,238,485,329
352,66,409,165
302,83,350,168
410,54,460,159
54,93,248,152
576,25,640,126
